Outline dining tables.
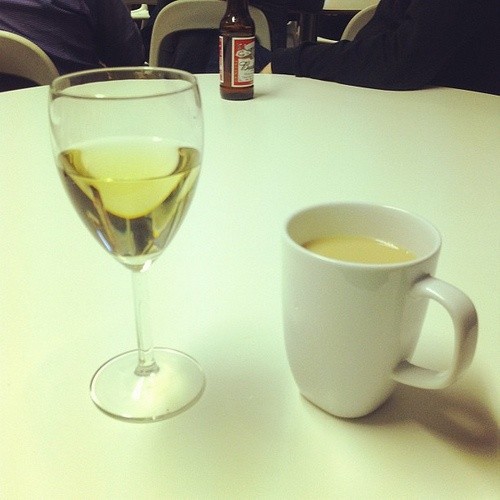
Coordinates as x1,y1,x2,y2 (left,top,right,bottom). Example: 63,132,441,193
0,71,500,498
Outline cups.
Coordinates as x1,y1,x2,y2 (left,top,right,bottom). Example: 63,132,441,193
284,202,478,418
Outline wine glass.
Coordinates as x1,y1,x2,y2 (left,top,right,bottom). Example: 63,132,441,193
46,68,205,419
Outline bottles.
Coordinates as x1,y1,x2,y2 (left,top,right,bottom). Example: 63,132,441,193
219,0,255,99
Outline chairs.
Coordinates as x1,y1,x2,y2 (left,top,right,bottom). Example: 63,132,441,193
133,0,272,73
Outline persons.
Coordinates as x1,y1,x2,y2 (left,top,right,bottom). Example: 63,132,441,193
258,0,500,97
0,0,147,93
